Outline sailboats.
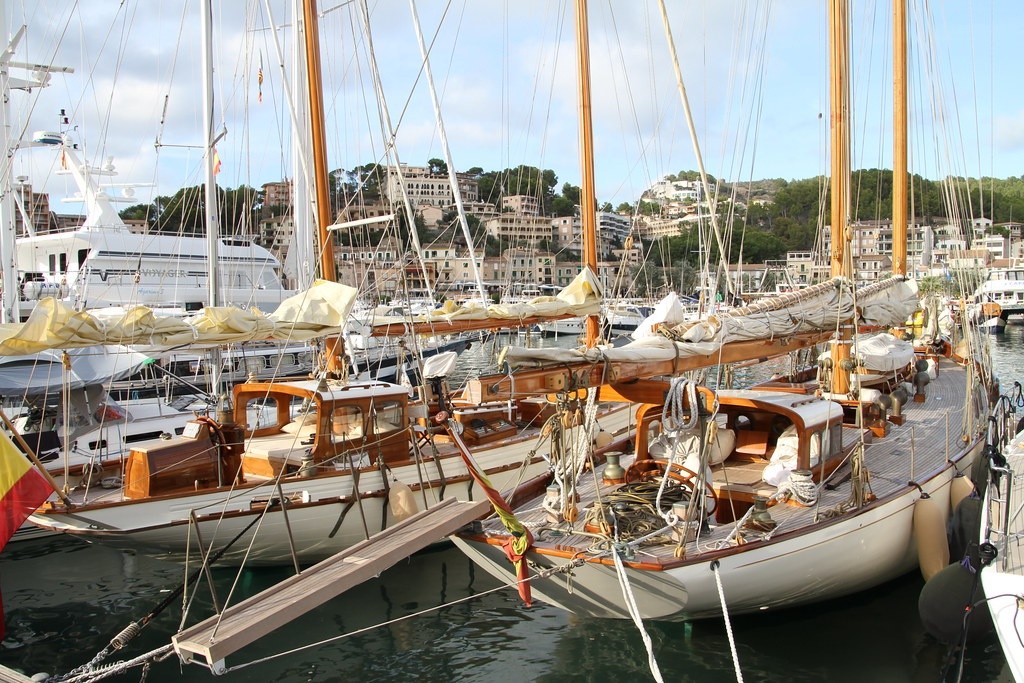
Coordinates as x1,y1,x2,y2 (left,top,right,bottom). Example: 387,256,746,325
0,0,1024,683
